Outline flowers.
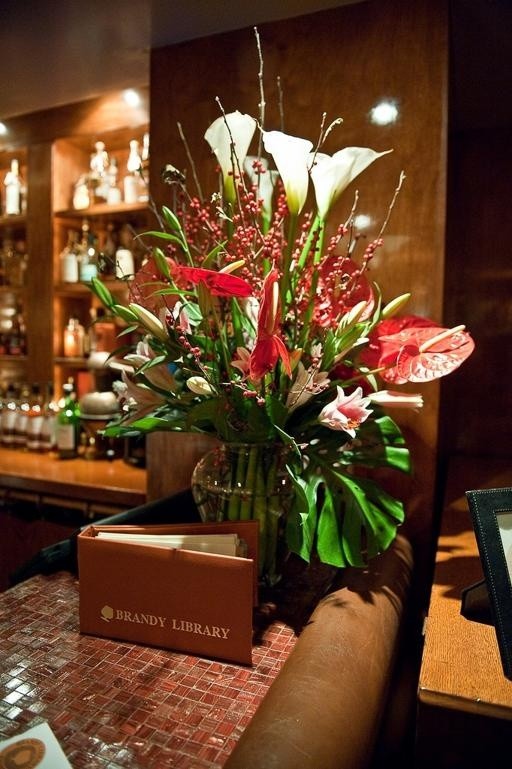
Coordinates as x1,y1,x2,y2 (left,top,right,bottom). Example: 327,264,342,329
60,24,479,573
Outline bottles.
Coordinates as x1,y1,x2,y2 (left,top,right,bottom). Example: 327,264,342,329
140,136,149,201
1,378,80,458
91,141,111,202
63,297,86,357
122,139,140,202
4,155,26,215
59,219,137,283
9,315,25,356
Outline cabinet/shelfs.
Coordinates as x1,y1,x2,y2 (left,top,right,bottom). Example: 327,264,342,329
49,121,150,441
0,147,31,405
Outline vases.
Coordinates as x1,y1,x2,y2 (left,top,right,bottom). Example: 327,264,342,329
190,442,303,588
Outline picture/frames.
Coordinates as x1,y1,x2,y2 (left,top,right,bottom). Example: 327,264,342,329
460,488,512,681
